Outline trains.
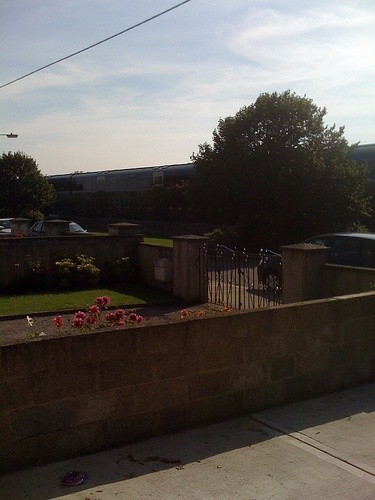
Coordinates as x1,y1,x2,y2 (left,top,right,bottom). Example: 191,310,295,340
45,150,375,221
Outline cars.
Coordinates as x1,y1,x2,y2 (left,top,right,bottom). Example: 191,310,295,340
256,233,375,291
1,219,88,235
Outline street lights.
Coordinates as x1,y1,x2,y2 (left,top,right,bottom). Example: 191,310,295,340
0,132,19,138
